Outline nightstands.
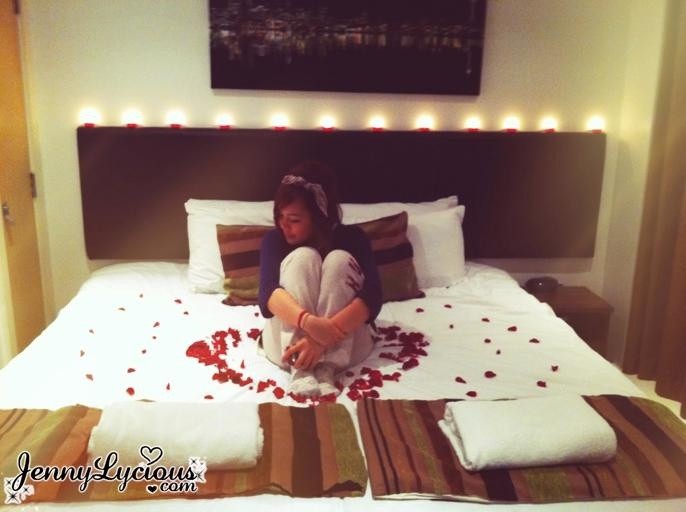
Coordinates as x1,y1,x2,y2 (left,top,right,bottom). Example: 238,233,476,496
522,285,617,360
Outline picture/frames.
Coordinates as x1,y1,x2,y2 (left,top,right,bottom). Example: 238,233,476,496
206,0,486,97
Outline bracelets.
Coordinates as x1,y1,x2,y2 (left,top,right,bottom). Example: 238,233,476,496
300,312,311,329
296,309,308,329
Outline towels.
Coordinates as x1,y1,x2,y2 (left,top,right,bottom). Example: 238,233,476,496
435,393,622,473
86,401,266,472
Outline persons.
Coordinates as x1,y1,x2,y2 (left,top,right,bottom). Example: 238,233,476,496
256,158,383,401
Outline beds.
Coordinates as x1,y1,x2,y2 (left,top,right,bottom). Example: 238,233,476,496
0,128,686,511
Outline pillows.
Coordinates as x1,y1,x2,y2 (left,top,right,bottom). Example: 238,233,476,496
182,191,472,307
